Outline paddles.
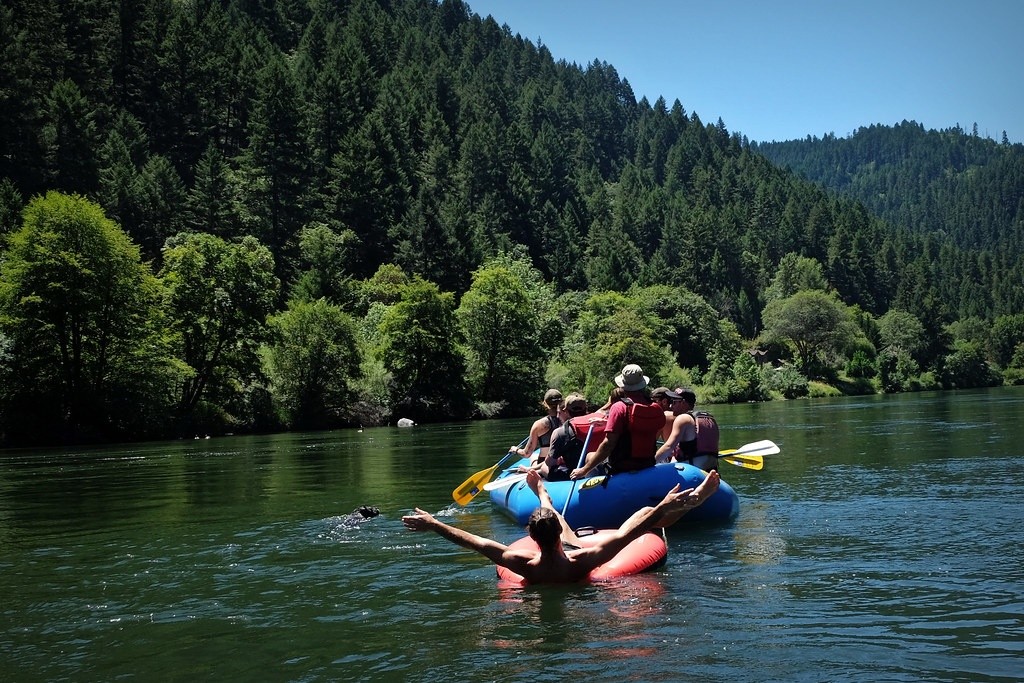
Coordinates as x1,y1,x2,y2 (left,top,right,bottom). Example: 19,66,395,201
718,439,781,461
655,440,764,471
560,423,594,516
452,434,532,505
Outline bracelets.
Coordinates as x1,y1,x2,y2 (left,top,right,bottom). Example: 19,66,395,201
516,449,520,454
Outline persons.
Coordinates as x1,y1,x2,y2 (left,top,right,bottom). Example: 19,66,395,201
508,363,721,480
401,469,720,588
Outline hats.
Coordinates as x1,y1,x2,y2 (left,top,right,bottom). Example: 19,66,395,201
649,387,672,399
544,389,563,405
562,391,586,413
614,364,650,392
665,387,696,403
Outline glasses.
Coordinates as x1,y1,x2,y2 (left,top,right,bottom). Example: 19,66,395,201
673,399,682,404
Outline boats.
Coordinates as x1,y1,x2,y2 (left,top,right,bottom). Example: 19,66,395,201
486,440,742,526
